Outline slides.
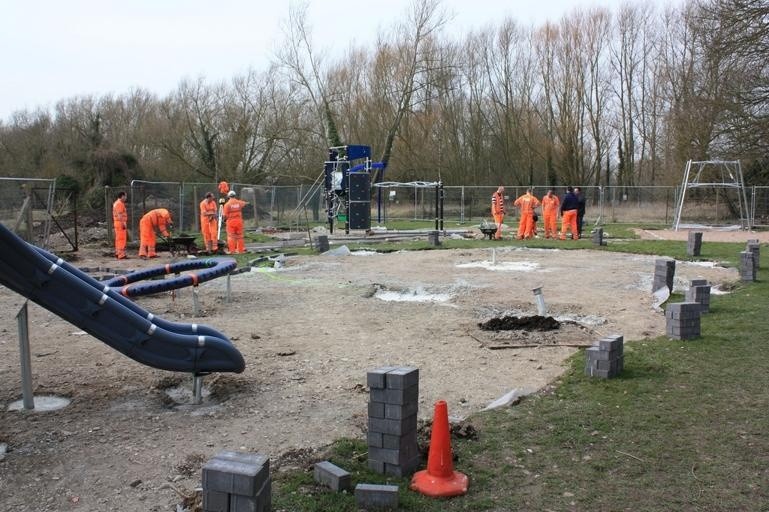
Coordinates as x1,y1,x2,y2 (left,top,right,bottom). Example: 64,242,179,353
0,223,245,373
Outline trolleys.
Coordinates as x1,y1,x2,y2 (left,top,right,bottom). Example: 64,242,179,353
478,218,497,240
157,227,199,256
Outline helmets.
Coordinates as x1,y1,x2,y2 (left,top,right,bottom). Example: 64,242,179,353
228,191,235,197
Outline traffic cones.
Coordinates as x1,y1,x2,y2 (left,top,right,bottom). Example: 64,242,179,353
410,399,469,496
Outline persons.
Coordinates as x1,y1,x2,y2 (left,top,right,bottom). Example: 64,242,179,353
113,192,130,259
200,192,219,255
491,186,507,239
514,186,585,240
221,190,247,254
139,207,174,259
